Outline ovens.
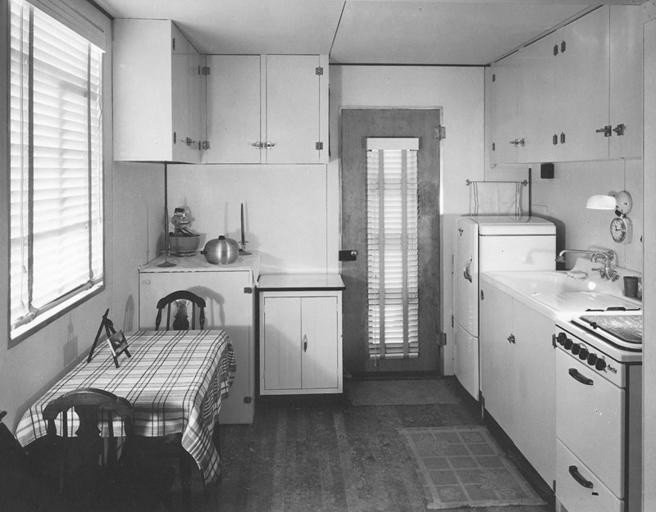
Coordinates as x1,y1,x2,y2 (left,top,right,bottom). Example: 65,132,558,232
551,324,628,511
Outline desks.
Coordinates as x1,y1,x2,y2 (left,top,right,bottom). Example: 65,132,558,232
17,327,232,512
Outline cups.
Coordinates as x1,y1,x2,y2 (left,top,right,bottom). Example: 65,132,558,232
623,276,638,298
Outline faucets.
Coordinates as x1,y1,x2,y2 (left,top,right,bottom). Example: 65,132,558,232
557,248,608,264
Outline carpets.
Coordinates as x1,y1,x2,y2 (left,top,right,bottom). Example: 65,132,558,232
349,379,460,405
401,422,548,510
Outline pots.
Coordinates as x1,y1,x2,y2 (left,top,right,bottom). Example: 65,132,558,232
199,234,244,264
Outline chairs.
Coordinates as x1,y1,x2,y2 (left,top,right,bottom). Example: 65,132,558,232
154,290,206,329
41,388,155,511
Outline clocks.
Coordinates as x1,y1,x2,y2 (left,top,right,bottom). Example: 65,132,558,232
610,215,632,244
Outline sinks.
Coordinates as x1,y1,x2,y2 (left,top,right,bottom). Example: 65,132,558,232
484,269,593,293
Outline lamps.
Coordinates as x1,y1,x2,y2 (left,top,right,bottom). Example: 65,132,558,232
586,192,632,214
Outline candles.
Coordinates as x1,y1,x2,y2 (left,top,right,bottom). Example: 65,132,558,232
164,210,168,249
241,204,245,242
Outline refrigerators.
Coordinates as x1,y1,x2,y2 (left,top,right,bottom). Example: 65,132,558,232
452,212,557,417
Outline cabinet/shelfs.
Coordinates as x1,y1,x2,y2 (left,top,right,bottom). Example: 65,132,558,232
204,55,330,163
114,18,207,163
259,292,347,401
483,8,642,166
138,270,258,425
